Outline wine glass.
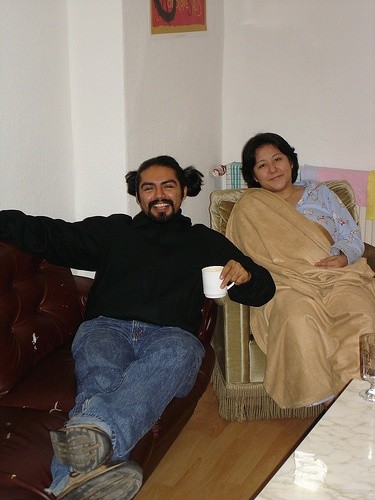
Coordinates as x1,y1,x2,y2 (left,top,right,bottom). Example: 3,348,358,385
359,333,375,402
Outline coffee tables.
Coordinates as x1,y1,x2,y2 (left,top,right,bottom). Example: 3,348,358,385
249,379,374,499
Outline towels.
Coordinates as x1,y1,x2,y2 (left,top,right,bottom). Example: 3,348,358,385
226,161,250,190
300,165,317,181
365,171,375,220
316,166,367,207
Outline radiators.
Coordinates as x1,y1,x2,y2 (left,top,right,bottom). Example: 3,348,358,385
227,163,375,247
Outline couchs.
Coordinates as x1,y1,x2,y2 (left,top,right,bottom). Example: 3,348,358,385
209,181,360,420
1,210,218,500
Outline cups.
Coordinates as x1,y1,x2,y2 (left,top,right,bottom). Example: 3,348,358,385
202,265,235,299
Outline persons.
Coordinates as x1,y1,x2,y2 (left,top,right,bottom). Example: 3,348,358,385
0,156,277,500
226,132,375,409
